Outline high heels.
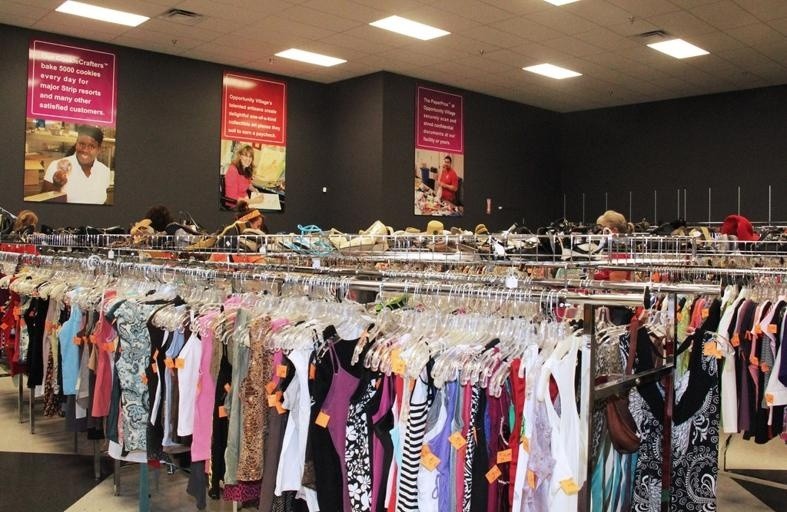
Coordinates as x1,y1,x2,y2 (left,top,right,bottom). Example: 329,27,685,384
329,220,389,256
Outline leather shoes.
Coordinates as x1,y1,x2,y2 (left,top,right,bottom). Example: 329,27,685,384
238,228,294,254
174,228,217,251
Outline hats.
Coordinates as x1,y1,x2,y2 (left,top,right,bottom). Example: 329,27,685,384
722,216,760,241
146,206,176,232
421,220,451,237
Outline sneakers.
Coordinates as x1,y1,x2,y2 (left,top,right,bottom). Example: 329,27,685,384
166,221,197,234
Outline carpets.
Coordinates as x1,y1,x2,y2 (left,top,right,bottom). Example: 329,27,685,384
0,449,116,511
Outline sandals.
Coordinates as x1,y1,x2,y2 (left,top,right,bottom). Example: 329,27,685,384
280,225,336,256
453,222,536,260
537,225,610,260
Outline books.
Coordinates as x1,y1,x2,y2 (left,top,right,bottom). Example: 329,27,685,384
248,190,282,212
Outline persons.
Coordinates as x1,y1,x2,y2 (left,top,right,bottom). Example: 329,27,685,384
434,155,460,205
141,205,179,231
594,209,634,236
227,208,267,238
222,145,264,210
12,207,40,240
42,124,114,203
